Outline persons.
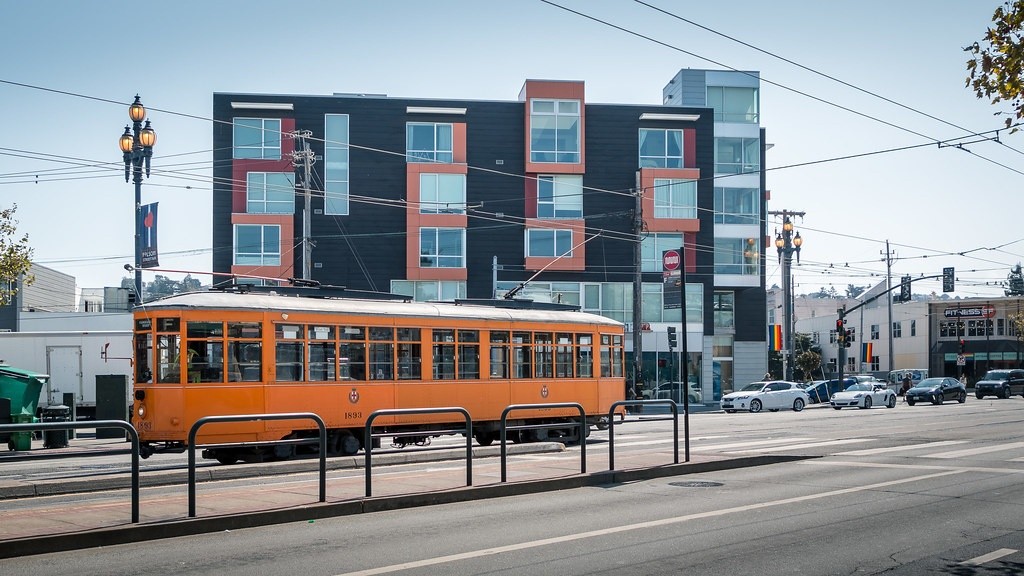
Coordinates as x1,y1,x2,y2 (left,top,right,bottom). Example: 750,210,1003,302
902,372,914,402
959,373,967,388
762,373,771,381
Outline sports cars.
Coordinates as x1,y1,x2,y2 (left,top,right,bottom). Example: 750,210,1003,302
831,381,896,409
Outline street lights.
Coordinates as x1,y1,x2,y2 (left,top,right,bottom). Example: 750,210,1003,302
776,215,803,381
118,93,157,307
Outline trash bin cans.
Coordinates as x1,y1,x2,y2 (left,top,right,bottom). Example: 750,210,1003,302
8,414,33,451
40,405,70,447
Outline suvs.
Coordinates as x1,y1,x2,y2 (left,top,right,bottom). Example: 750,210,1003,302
975,369,1024,399
642,382,703,403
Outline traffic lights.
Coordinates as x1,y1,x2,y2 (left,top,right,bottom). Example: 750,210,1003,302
959,339,966,354
844,330,851,347
668,326,678,349
837,320,842,332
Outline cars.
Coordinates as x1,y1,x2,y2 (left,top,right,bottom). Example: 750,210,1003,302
904,377,967,405
848,375,877,389
804,379,856,405
720,380,810,414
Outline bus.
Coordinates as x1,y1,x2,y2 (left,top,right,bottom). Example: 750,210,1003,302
128,289,628,462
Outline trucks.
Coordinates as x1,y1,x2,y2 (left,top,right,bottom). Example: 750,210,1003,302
888,369,928,395
